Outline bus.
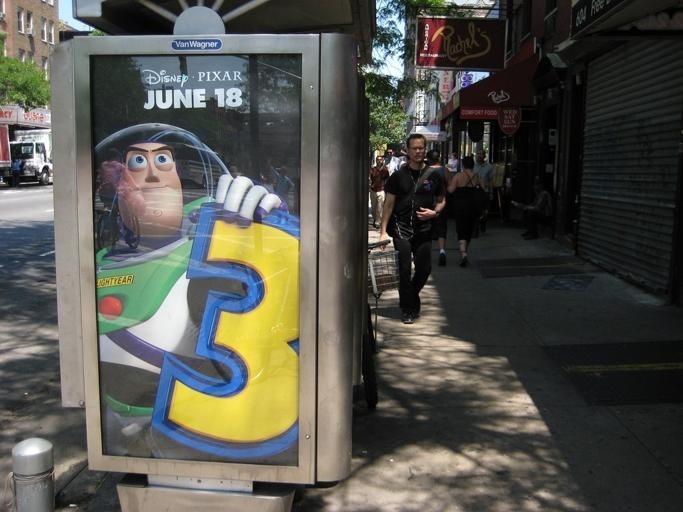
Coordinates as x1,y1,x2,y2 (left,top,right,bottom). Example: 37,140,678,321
165,141,223,189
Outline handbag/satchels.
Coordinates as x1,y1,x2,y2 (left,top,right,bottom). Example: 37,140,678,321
386,210,413,240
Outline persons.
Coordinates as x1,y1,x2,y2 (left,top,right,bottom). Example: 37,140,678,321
377,131,446,324
10,153,25,188
517,174,553,242
370,145,498,269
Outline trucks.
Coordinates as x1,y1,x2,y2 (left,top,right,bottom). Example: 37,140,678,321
2,128,53,187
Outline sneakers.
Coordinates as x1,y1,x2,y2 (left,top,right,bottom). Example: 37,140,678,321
438,249,468,267
401,295,420,324
522,232,538,240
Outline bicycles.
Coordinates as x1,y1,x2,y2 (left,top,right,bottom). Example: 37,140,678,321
96,184,143,257
359,239,402,413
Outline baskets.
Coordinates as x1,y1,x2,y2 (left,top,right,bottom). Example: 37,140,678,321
367,250,400,288
